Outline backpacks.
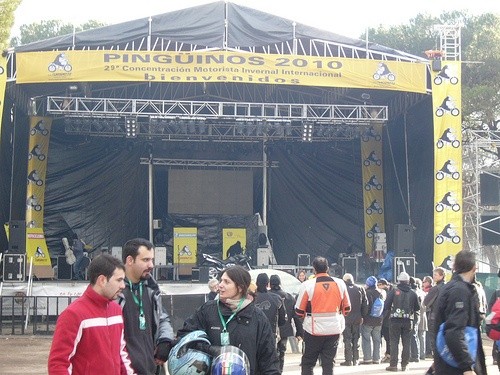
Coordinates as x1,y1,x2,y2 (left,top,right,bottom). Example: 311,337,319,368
369,298,384,318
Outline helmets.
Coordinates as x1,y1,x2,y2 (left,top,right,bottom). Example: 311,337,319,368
167,331,211,375
208,344,251,375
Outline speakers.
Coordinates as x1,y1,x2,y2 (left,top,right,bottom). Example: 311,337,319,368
393,224,414,257
8,220,26,254
57,254,88,281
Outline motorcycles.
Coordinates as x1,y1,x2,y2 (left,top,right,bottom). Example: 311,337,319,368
199,245,253,281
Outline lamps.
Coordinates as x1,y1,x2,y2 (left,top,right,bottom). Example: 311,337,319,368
62,110,370,141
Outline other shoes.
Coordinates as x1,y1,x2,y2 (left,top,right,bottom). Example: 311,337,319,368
380,356,391,363
420,356,426,360
359,360,371,364
386,365,397,371
425,354,434,358
372,360,380,364
339,361,353,366
354,360,360,367
408,357,420,362
401,366,406,371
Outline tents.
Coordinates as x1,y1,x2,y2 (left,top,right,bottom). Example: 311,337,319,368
0,0,433,282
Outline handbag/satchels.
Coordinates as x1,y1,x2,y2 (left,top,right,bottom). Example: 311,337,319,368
434,323,478,369
278,323,294,338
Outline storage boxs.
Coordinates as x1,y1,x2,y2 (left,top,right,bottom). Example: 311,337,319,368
340,253,359,280
392,256,416,285
2,253,25,283
297,253,311,274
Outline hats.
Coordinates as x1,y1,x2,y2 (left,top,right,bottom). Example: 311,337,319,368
270,274,282,285
256,272,270,287
342,273,355,285
366,276,378,288
396,272,410,282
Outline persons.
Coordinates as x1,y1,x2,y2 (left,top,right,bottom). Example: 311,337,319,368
359,276,383,363
297,270,308,282
410,267,446,363
485,269,500,371
226,241,243,258
253,273,283,343
209,278,219,300
471,273,487,375
48,254,139,375
176,265,281,375
294,256,351,375
428,249,488,375
268,275,296,374
114,238,174,375
340,273,368,366
282,321,300,353
378,271,420,372
